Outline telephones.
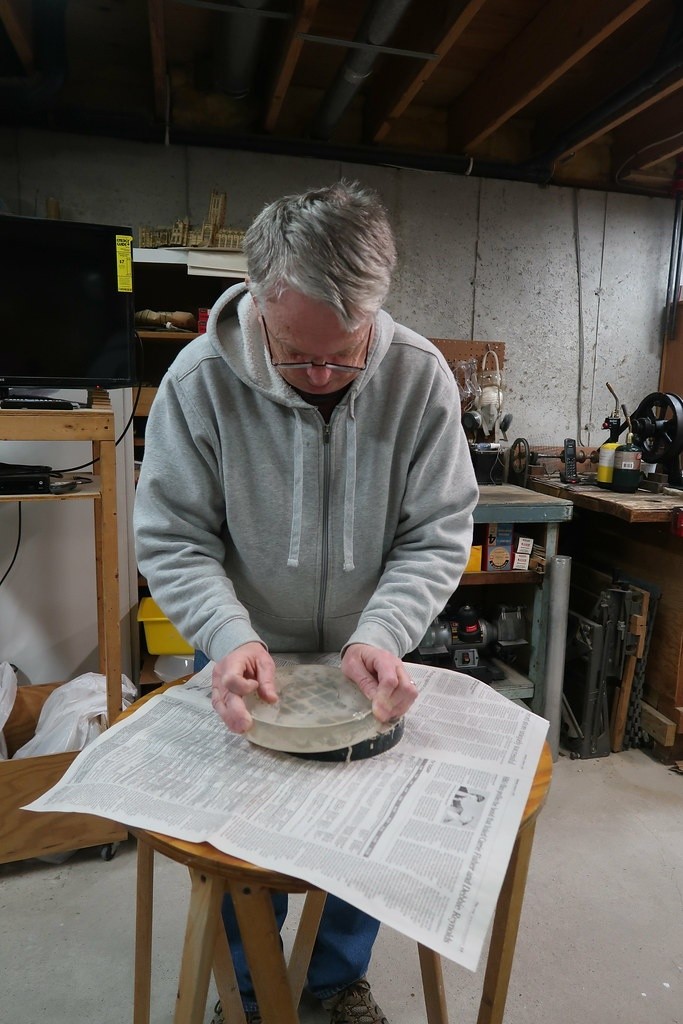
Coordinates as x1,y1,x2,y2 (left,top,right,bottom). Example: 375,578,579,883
560,437,581,485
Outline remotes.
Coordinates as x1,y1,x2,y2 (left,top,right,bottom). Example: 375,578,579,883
0,399,73,410
49,481,78,494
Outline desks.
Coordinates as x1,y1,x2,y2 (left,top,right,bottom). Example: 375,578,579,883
535,474,683,764
110,673,554,1024
474,483,574,764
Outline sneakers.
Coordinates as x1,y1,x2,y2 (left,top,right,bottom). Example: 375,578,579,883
210,999,262,1024
321,975,389,1024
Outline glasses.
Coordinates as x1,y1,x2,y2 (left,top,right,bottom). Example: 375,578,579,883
260,310,373,373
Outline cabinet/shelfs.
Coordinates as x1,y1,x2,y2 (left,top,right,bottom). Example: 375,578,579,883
133,332,204,688
0,388,131,869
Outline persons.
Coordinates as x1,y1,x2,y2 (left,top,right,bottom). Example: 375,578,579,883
133,185,480,1024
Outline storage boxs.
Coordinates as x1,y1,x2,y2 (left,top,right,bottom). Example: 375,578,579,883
136,596,195,655
482,546,514,572
464,546,483,573
475,523,515,545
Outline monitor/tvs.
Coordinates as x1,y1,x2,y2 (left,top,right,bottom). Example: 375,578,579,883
0,213,138,396
